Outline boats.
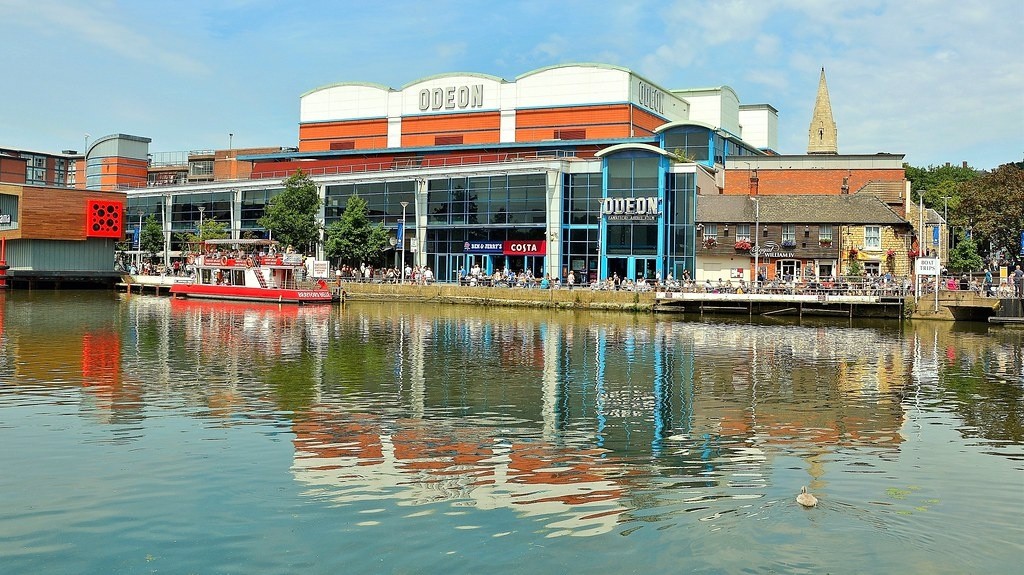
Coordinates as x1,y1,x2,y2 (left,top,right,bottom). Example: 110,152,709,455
168,237,333,307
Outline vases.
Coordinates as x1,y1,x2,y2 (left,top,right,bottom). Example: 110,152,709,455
706,244,714,249
782,245,796,250
735,248,750,254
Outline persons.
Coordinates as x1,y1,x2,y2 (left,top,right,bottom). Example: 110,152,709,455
207,245,327,289
1014,265,1024,297
127,259,185,277
982,248,1023,271
330,262,436,285
1009,271,1016,298
566,270,575,290
984,269,995,297
580,264,587,287
589,268,982,298
458,264,560,290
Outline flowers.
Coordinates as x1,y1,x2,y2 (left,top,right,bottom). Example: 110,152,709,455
733,239,752,250
909,249,915,260
848,249,858,260
887,249,896,256
780,237,797,246
702,236,717,246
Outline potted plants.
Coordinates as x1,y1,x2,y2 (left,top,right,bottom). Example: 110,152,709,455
819,238,832,248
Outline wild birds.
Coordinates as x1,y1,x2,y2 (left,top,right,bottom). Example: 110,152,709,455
796,486,819,506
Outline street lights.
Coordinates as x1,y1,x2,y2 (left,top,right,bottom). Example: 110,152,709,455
596,198,606,283
750,196,761,287
228,133,234,148
136,209,144,250
400,200,410,283
915,189,928,318
82,133,91,155
198,206,206,254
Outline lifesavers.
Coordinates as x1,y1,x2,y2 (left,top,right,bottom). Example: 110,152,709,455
220,255,228,266
187,254,196,265
245,257,253,268
